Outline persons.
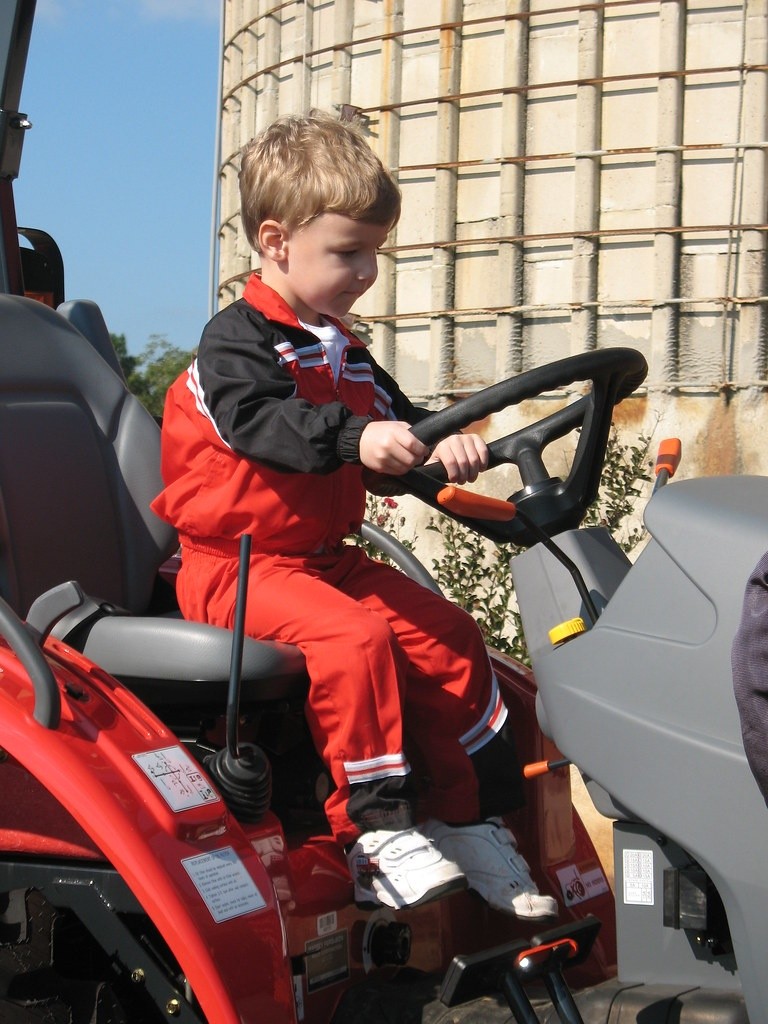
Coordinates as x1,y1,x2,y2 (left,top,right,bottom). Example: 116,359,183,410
149,115,558,920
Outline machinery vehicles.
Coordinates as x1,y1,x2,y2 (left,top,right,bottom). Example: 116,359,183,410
3,222,768,1024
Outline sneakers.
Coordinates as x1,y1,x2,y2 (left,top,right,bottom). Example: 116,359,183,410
343,829,469,911
416,817,558,921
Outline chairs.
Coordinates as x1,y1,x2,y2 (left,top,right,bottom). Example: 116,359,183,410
55,299,129,389
0,294,446,703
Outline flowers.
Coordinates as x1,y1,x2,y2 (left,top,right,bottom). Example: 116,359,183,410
345,494,418,567
